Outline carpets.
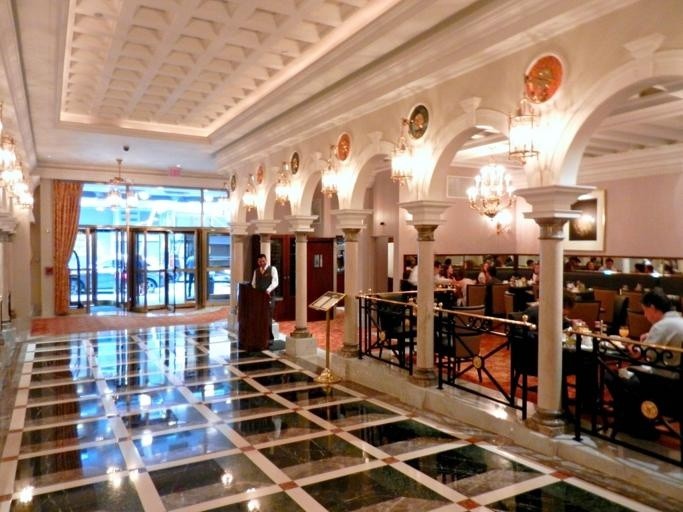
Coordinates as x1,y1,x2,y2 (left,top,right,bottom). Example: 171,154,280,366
29,303,230,337
275,307,682,451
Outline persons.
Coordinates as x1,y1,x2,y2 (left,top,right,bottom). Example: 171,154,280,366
520,287,576,334
625,289,682,366
249,253,280,347
405,254,678,291
183,249,195,299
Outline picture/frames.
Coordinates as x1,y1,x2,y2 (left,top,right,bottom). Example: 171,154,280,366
562,188,607,251
446,175,475,200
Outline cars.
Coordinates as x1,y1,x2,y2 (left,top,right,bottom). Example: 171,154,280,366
68,253,173,294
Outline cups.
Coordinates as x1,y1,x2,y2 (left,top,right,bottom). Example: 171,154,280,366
617,325,629,338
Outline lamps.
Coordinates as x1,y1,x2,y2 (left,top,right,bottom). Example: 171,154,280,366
313,253,323,269
321,144,345,199
390,118,419,187
103,159,140,211
242,176,258,213
0,102,35,209
274,161,292,206
466,74,553,235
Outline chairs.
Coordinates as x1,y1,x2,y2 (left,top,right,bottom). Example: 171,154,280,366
376,282,683,438
